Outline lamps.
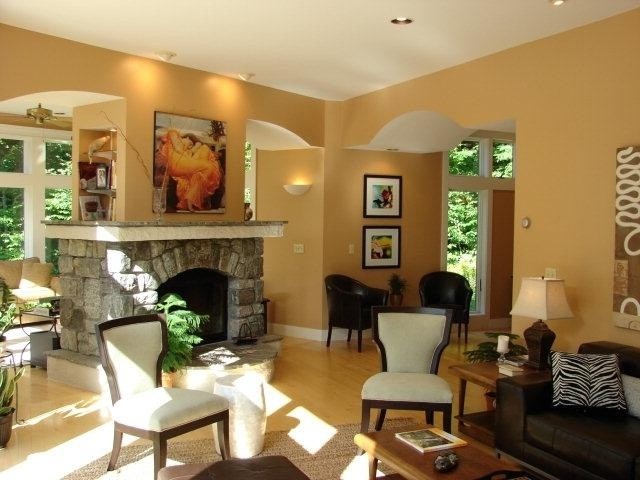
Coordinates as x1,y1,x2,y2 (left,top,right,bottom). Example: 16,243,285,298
508,277,575,374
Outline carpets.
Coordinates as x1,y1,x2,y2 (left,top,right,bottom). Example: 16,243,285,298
60,417,560,479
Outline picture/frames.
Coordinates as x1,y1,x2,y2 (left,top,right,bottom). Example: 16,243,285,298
152,111,229,215
361,224,401,271
361,173,403,218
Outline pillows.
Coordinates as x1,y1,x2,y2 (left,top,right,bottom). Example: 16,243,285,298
1,254,40,288
549,351,630,413
17,262,55,291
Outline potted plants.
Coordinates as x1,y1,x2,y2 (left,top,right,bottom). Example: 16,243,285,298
150,292,212,389
462,329,530,411
0,362,26,451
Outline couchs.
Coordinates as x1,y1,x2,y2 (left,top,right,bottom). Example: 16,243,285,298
0,256,62,327
491,340,640,479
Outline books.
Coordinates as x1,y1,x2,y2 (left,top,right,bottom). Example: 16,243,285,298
395,426,467,455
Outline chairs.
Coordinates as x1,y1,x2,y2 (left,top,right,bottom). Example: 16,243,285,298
324,273,392,354
361,304,453,457
94,313,232,480
417,271,476,346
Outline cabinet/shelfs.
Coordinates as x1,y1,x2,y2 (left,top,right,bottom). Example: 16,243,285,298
448,359,551,450
